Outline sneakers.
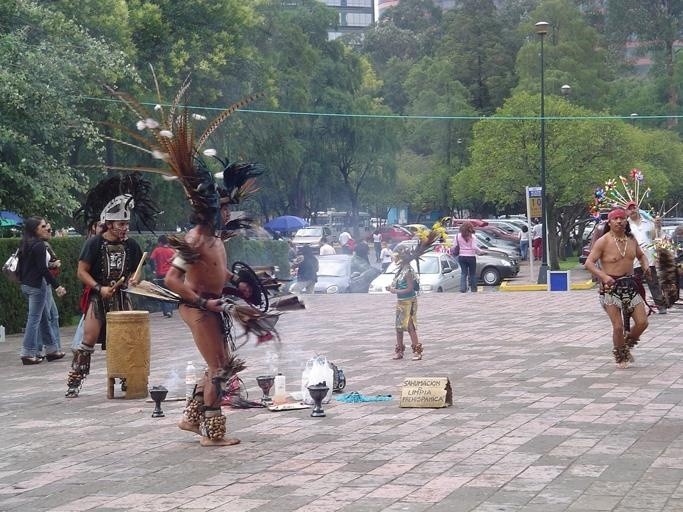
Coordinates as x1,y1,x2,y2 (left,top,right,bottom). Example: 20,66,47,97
412,353,422,360
393,351,405,360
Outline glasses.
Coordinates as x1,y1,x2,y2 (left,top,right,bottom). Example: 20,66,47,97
48,229,54,233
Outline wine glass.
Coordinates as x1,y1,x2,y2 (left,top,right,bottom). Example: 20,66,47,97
306,380,330,417
256,374,274,406
149,389,169,418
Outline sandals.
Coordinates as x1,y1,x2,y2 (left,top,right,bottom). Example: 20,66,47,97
66,386,79,398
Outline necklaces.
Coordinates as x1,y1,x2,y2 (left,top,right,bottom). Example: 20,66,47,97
612,235,627,257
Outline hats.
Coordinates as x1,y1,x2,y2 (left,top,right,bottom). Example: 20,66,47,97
100,194,134,224
626,202,637,209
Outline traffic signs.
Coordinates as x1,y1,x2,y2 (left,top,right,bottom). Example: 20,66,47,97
530,197,542,219
529,187,542,197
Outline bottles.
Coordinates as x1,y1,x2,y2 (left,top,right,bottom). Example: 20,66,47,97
183,360,196,396
273,372,286,403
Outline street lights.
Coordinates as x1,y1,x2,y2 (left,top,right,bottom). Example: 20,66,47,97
561,83,571,99
630,112,640,125
535,19,551,284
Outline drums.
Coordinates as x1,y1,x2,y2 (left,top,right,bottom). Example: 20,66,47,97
106,310,149,398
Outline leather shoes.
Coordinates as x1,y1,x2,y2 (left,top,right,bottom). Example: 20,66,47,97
21,350,65,364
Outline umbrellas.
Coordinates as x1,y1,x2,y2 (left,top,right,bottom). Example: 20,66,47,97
0,210,25,238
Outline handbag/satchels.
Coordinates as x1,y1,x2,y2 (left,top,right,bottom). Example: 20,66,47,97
48,260,61,278
2,254,21,281
450,244,459,256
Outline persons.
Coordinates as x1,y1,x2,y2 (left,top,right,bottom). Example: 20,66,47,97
150,235,175,317
164,202,253,448
533,219,543,260
384,250,423,362
453,218,488,293
53,224,84,237
64,220,145,399
295,244,320,294
36,219,64,358
69,220,103,374
319,238,336,255
339,229,383,264
583,207,652,369
518,225,529,261
18,216,66,365
624,200,666,314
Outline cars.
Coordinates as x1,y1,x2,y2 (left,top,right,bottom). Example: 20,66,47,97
368,251,469,292
286,207,534,285
290,255,380,295
65,226,80,235
176,220,280,242
534,216,682,265
